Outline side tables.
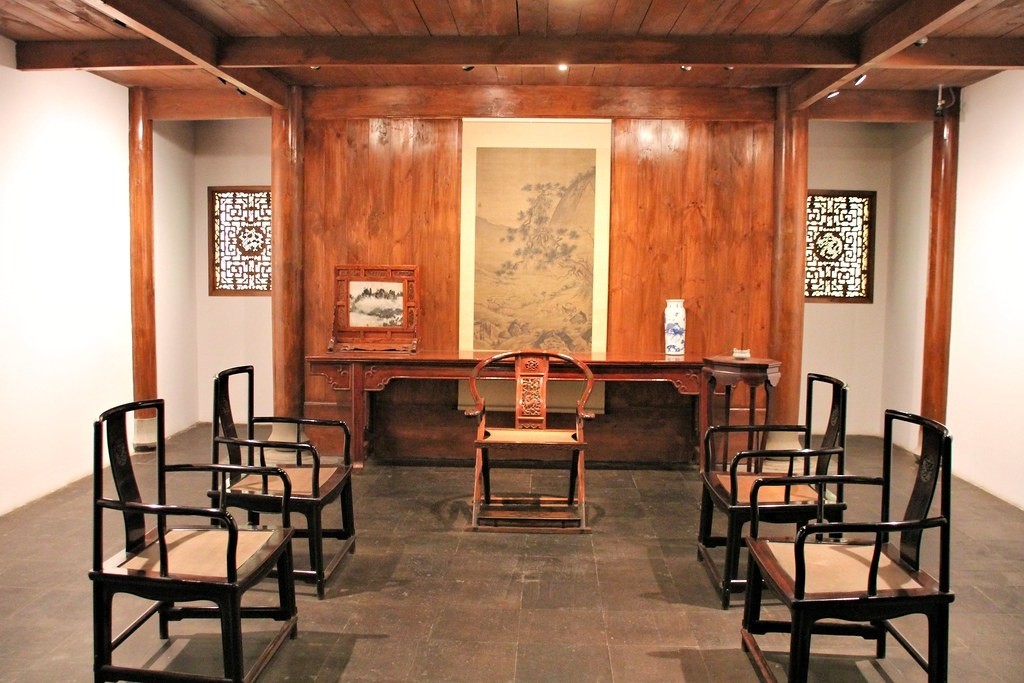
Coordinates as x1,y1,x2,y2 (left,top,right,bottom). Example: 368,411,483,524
699,355,782,473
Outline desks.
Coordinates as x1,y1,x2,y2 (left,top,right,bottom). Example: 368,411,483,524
307,349,739,475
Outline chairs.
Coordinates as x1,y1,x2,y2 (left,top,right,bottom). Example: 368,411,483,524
699,372,849,609
463,350,596,533
739,410,955,683
207,366,356,599
88,400,298,683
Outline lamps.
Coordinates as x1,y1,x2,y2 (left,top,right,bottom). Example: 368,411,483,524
828,91,839,99
855,74,866,86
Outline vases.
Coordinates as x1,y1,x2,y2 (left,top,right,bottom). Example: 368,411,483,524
665,298,686,355
